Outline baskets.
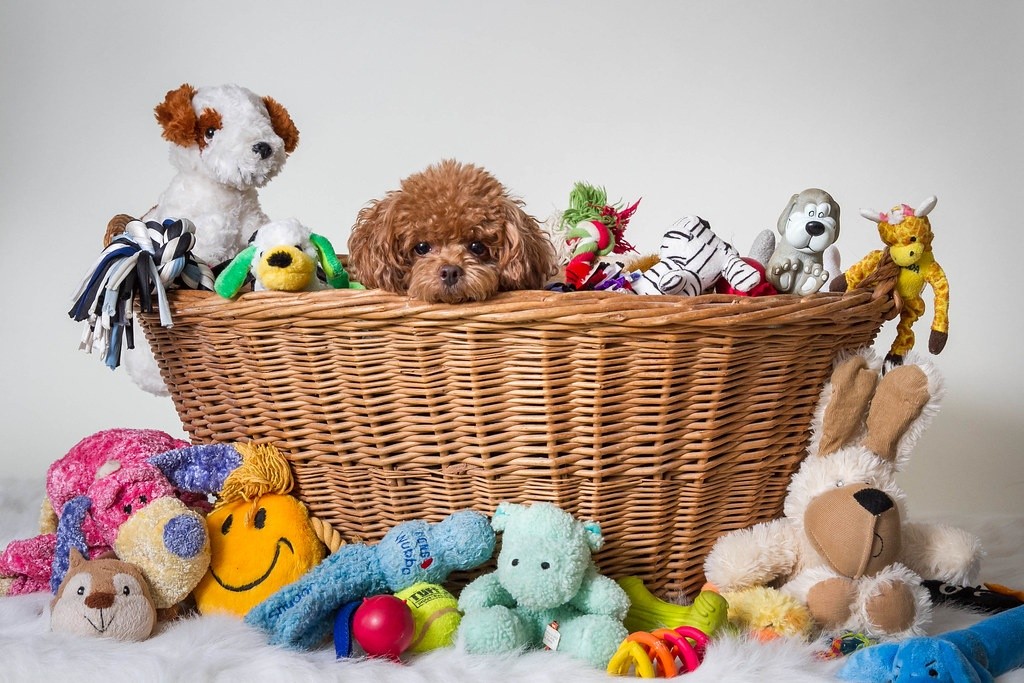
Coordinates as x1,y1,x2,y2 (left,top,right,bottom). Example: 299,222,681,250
103,214,904,606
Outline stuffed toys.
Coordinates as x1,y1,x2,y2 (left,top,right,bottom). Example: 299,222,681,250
0,79,1024,683
